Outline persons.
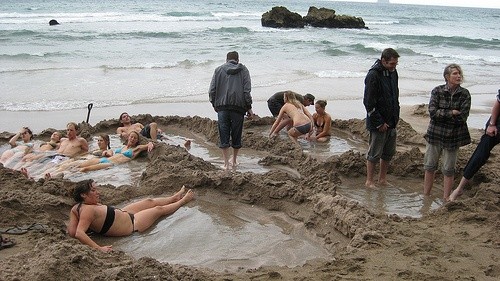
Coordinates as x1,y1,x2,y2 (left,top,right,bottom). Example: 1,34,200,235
363,48,401,191
447,89,500,203
67,178,195,254
423,63,472,199
304,100,332,143
268,90,315,147
208,51,254,171
267,90,315,134
0,122,155,181
116,112,192,150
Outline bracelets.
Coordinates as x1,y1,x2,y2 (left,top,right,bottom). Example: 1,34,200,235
489,122,496,127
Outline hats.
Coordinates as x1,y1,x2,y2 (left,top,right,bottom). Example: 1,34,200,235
305,93,315,105
